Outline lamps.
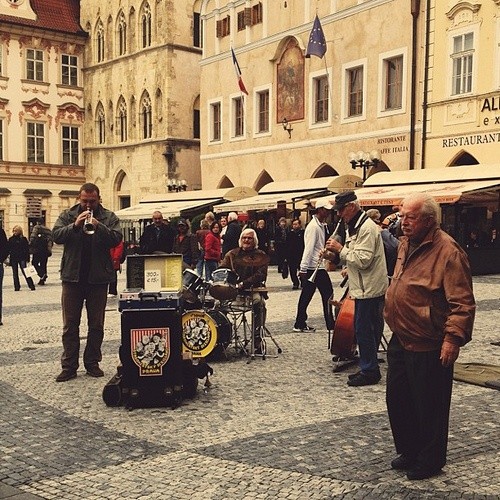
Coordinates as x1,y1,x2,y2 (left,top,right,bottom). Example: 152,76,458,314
282,117,293,138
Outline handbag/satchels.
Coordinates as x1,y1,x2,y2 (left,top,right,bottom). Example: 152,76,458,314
282,261,288,279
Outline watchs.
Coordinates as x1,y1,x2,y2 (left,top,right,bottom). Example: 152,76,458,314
240,282,245,288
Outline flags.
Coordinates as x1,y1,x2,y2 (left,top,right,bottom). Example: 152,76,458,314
231,47,249,95
306,15,327,59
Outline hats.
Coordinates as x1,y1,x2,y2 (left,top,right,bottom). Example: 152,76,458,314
332,191,357,209
315,198,334,210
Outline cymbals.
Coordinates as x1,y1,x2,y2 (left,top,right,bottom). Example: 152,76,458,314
244,286,281,291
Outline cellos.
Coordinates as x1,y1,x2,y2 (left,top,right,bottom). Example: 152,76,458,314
328,213,399,373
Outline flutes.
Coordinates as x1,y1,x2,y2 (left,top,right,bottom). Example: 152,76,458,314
308,218,343,284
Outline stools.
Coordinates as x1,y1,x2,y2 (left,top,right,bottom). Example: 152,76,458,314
227,307,255,356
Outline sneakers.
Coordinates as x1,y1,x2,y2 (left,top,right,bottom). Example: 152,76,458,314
293,325,316,333
407,462,440,480
391,453,416,469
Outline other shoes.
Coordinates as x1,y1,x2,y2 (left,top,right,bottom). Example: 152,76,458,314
56,369,76,381
347,372,381,387
107,294,116,298
87,365,104,377
253,337,262,351
38,275,47,285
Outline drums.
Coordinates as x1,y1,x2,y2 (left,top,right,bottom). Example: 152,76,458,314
180,307,232,363
181,270,204,303
209,267,238,301
219,295,252,314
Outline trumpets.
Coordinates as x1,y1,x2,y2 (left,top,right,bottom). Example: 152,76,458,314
84,207,96,235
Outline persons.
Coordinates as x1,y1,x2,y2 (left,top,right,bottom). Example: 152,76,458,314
0,217,54,325
109,240,125,295
442,222,485,249
124,211,272,291
220,229,270,354
52,183,123,382
318,191,403,386
383,193,476,480
293,199,336,333
274,217,304,289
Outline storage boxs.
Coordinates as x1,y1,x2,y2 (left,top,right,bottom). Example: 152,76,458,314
117,253,184,313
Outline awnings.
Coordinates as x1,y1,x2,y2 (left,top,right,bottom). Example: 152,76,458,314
214,174,364,212
309,163,500,208
115,186,259,220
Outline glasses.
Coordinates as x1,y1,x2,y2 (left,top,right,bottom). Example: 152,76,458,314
243,236,252,239
